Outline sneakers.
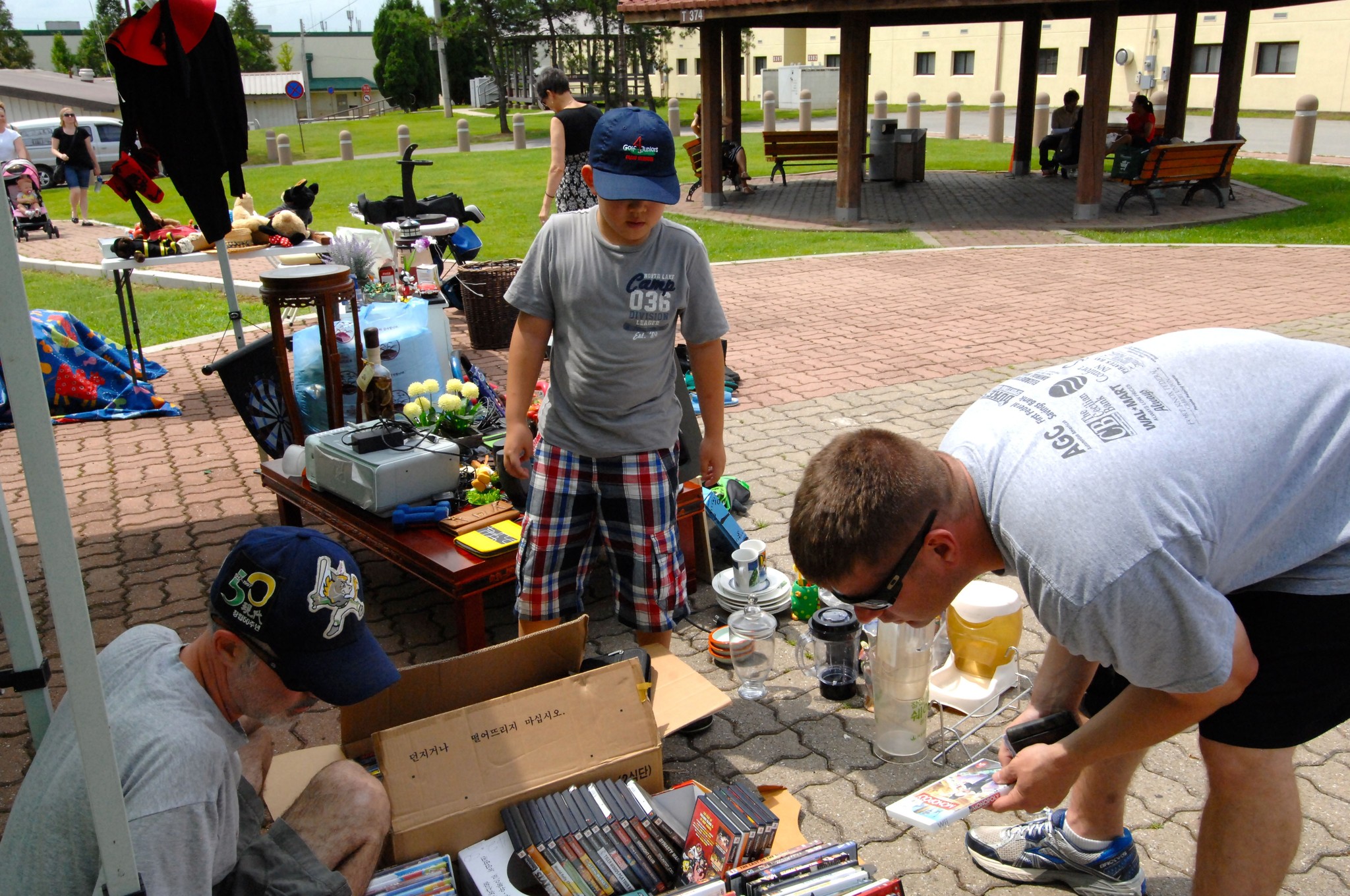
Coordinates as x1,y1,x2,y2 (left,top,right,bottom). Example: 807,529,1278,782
966,805,1146,896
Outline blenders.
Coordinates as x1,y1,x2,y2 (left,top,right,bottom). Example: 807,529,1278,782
795,606,864,701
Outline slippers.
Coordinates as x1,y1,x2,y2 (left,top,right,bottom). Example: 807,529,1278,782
724,392,740,407
688,393,703,416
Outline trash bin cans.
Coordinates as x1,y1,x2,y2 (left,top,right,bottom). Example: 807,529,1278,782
869,119,898,182
893,129,927,182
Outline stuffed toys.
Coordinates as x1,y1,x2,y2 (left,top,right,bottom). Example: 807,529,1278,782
111,178,319,262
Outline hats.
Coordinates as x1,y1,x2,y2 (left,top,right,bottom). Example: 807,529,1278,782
211,530,400,708
589,108,680,204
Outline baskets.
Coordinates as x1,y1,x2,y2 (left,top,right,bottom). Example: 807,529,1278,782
457,259,526,350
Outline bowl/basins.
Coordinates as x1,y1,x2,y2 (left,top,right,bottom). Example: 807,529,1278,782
708,625,755,664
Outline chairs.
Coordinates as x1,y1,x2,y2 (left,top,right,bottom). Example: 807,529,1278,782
265,250,325,328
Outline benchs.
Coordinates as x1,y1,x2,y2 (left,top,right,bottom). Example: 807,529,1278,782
682,137,740,202
761,129,875,188
1104,123,1247,217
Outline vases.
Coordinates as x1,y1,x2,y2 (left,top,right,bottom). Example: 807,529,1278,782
443,425,484,454
398,282,416,296
354,277,372,291
362,292,397,305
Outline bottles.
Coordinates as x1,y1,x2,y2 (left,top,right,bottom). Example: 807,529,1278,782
94,176,103,192
362,327,395,421
859,620,878,713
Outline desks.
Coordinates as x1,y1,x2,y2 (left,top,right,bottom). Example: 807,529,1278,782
251,456,707,655
350,212,460,274
413,292,459,397
100,231,341,390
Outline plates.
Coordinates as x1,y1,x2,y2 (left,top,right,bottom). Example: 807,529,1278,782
712,567,792,614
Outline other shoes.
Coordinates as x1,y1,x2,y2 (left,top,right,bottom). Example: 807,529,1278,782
71,215,79,224
82,222,94,226
683,374,696,389
724,381,738,392
27,209,40,219
645,653,713,734
1042,169,1054,177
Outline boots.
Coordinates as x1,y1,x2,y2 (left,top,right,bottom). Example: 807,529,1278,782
673,343,693,374
720,338,741,380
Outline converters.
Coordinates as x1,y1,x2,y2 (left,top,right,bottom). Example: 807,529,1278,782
349,427,404,453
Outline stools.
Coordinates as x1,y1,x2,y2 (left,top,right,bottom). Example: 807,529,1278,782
259,263,365,447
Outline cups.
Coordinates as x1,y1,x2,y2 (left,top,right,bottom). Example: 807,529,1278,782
731,548,759,593
526,390,545,438
791,579,820,620
739,539,767,582
727,594,777,700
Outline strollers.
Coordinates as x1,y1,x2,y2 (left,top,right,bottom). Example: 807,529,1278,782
2,157,60,242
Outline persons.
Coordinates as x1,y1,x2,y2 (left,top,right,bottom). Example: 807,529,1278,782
535,65,603,225
1059,105,1084,182
502,107,730,733
1183,94,1240,144
789,328,1350,896
16,177,41,219
0,526,403,896
0,101,33,234
51,107,101,226
690,104,755,194
1105,95,1156,156
1039,89,1079,176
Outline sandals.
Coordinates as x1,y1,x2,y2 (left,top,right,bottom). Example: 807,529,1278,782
740,172,752,180
741,187,756,195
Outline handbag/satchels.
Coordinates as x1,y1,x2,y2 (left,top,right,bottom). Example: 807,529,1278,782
53,166,66,184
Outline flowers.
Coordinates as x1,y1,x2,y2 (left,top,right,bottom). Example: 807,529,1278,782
399,236,439,272
316,233,383,280
400,377,483,436
360,281,397,295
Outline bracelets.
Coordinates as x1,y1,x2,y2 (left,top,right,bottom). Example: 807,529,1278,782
1070,126,1073,130
545,191,554,198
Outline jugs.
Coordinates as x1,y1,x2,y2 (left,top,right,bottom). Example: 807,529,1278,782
868,618,933,765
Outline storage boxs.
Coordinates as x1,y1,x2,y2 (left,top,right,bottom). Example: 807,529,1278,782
98,237,122,259
257,610,734,869
701,486,749,551
460,780,727,896
414,263,441,287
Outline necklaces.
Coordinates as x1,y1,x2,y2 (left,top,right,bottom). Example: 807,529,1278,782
560,100,574,109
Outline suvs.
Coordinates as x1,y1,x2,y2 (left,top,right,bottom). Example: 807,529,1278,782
8,116,142,190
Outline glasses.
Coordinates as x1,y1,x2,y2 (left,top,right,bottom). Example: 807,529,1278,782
830,509,939,613
212,612,307,692
63,113,75,117
540,94,547,106
